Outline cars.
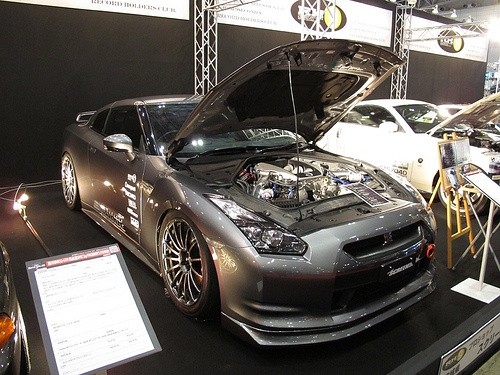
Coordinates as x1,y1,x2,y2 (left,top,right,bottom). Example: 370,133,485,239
0,241,31,375
61,38,439,350
315,92,500,218
437,103,500,146
